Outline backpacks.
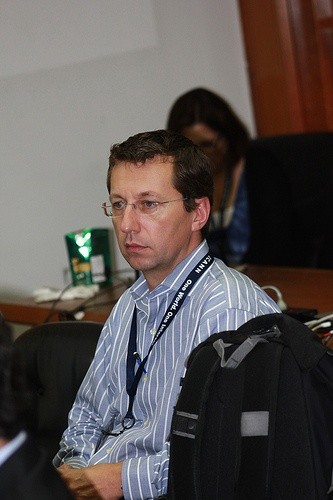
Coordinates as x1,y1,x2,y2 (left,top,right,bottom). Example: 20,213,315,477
168,313,333,500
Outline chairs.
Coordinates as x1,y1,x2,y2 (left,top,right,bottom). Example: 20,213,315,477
251,131,332,270
1,321,103,500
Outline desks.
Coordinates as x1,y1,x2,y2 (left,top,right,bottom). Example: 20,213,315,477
0,266,333,348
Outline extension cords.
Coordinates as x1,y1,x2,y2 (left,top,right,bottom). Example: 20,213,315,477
282,307,318,323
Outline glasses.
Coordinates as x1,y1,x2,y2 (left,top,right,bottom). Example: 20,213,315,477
102,197,188,218
203,134,222,154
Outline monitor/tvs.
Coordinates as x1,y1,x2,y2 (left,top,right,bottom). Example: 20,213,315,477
246,132,333,271
